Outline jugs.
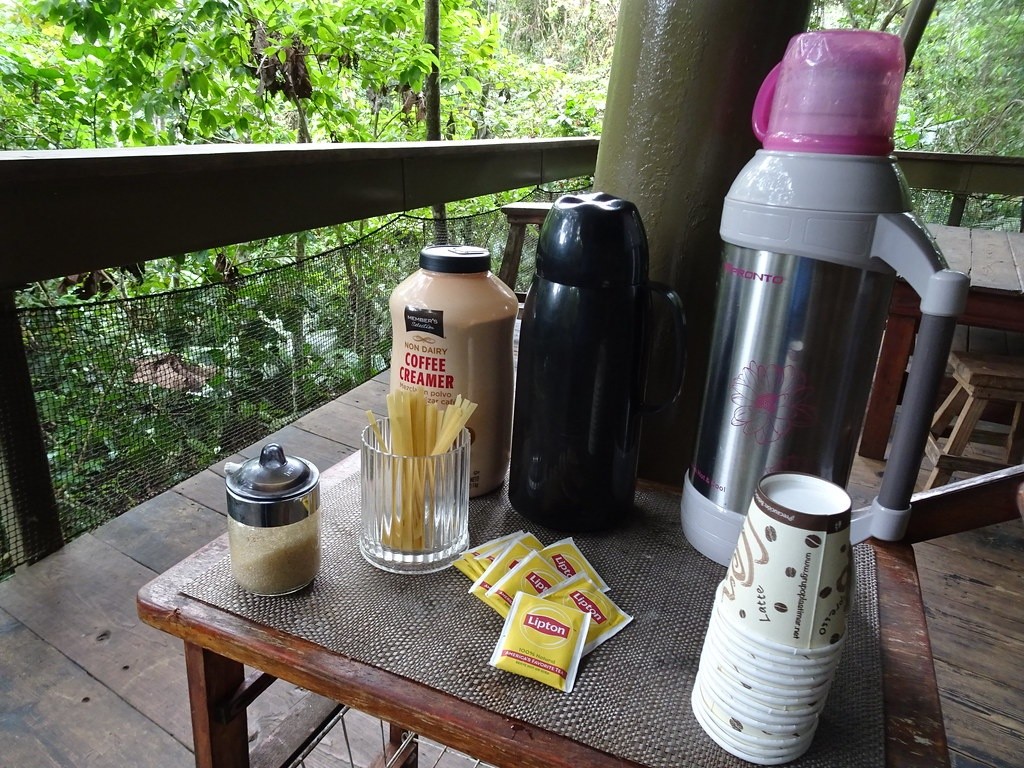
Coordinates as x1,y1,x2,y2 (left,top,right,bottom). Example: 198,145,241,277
683,31,971,568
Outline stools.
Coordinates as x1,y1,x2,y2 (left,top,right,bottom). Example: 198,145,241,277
922,351,1024,490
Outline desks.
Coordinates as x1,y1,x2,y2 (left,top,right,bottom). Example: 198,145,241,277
134,446,950,768
858,224,1024,461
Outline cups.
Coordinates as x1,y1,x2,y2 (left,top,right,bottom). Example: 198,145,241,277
362,419,471,575
691,472,850,765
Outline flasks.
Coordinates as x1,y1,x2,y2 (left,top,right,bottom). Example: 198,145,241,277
508,191,686,528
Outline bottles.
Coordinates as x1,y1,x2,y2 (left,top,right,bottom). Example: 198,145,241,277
224,443,322,596
388,247,519,497
